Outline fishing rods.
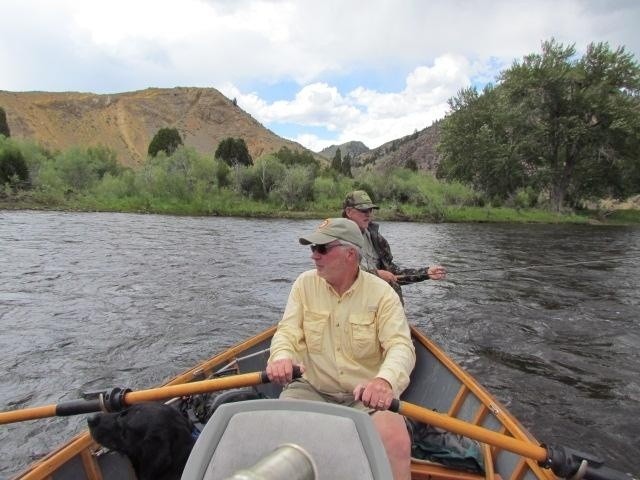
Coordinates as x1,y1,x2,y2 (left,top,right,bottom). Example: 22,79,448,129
395,256,640,280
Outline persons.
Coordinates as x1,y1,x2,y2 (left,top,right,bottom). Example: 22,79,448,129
338,190,444,310
265,217,416,480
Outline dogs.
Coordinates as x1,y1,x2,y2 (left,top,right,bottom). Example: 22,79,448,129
87,401,195,480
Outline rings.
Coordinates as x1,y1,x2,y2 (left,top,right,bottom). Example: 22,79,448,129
378,397,385,404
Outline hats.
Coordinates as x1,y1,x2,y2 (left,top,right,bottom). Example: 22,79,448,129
299,217,364,248
343,190,380,211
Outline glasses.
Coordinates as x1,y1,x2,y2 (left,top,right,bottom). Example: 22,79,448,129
311,244,345,255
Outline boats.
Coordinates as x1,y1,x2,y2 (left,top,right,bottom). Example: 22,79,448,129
9,320,562,479
